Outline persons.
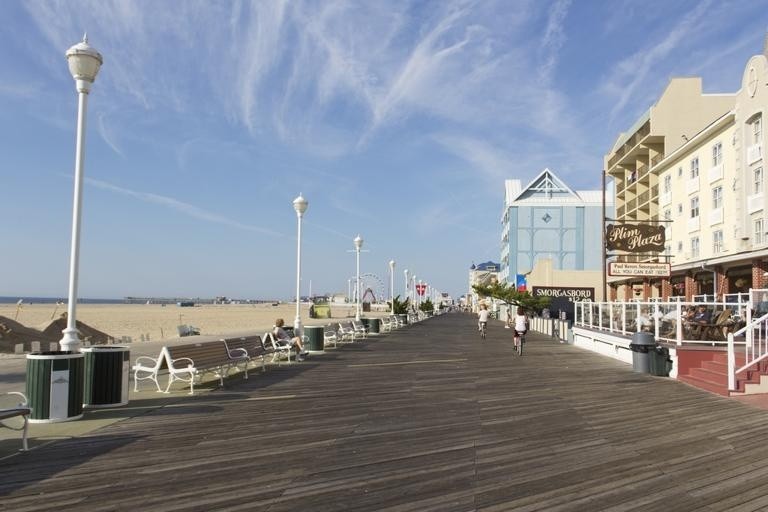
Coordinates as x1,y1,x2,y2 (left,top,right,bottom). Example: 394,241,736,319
512,306,528,350
644,304,711,339
273,319,309,361
478,304,489,334
505,309,511,328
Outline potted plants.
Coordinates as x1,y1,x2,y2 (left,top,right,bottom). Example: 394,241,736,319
419,297,434,318
386,294,410,324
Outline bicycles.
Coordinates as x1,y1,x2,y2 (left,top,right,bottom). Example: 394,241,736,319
510,322,529,356
480,322,487,339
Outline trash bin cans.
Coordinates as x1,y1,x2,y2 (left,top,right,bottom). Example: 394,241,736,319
303,326,324,353
649,346,669,377
632,332,656,373
24,350,85,424
360,318,379,335
78,345,130,409
273,325,294,331
390,314,407,325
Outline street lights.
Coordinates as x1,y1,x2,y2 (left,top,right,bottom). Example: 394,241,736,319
59,32,104,353
292,191,309,329
353,233,364,321
389,259,442,314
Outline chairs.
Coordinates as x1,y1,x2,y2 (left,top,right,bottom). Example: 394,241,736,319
380,315,404,331
0,391,32,456
131,319,372,395
